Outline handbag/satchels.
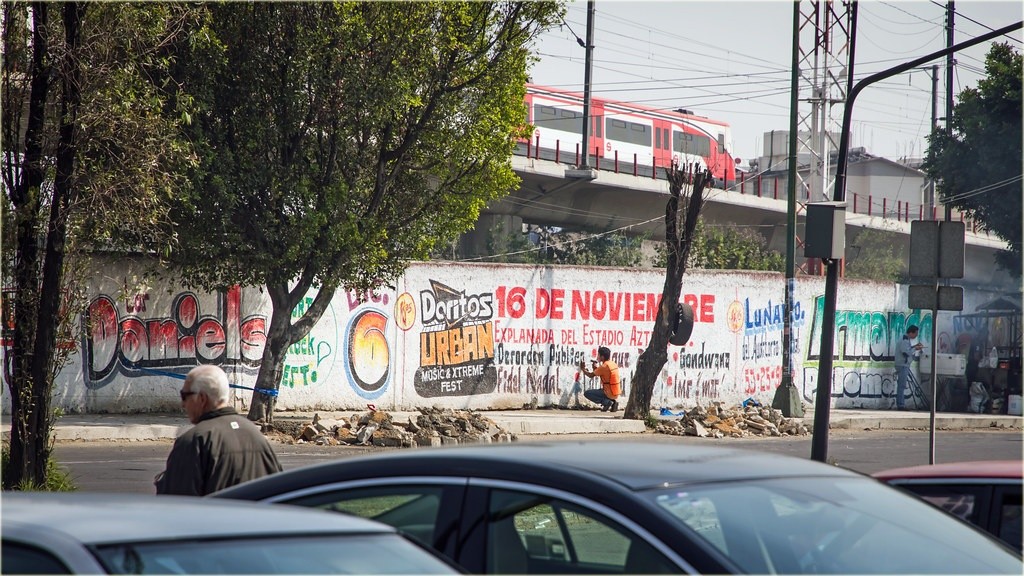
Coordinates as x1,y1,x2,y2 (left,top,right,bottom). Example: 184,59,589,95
989,347,998,369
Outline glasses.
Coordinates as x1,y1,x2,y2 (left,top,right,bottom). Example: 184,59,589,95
179,390,202,400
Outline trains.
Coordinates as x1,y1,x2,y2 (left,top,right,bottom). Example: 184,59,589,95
447,83,739,192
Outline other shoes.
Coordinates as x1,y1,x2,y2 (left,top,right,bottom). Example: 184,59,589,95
898,407,910,411
610,402,619,412
603,401,613,411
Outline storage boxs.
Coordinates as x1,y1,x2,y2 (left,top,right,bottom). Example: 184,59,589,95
919,353,968,377
1008,395,1023,416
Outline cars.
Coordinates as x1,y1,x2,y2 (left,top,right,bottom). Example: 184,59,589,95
204,435,1023,575
870,460,1022,554
1,492,480,576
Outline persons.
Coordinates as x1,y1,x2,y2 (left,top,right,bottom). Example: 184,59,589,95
895,325,923,411
156,364,283,498
581,347,620,412
